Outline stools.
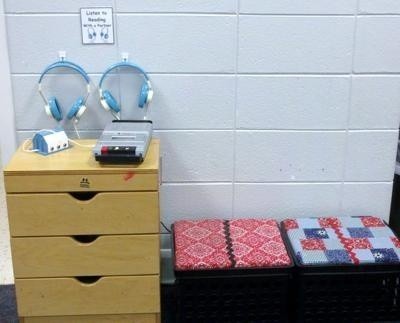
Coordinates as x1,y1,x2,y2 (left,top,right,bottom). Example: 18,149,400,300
167,214,399,322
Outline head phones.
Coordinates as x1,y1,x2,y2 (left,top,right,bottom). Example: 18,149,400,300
38,62,91,121
98,62,153,114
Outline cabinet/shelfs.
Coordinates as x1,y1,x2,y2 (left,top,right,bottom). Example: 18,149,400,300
2,136,164,323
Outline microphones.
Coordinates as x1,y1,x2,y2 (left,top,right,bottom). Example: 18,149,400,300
73,123,82,140
111,114,121,120
